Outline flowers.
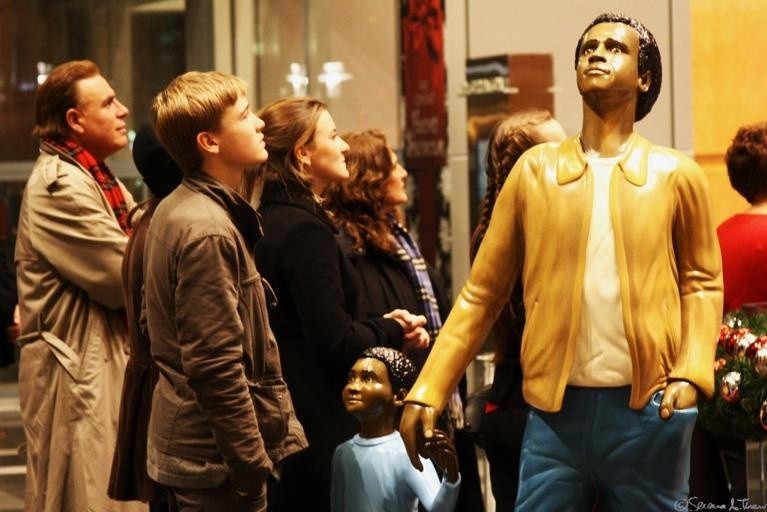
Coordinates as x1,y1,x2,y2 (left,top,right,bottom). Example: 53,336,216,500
698,305,767,443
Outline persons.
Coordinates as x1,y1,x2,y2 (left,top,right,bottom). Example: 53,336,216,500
398,9,727,512
254,93,434,511
329,343,463,511
11,56,151,512
104,120,186,511
142,67,311,511
465,101,574,510
329,123,488,510
714,114,767,328
